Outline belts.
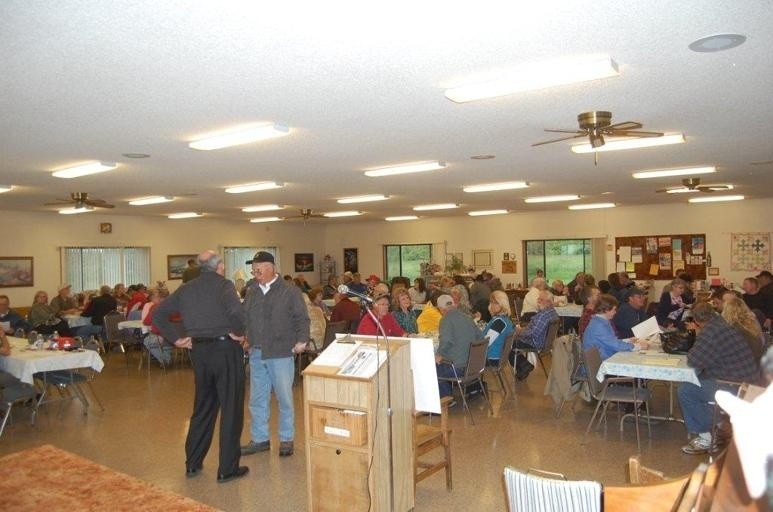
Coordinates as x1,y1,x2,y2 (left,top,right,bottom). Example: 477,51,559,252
191,335,231,342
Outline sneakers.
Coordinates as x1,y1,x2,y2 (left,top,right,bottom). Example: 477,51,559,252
516,363,535,380
448,400,457,408
240,441,270,455
467,382,489,401
682,424,732,454
626,409,660,425
279,441,294,454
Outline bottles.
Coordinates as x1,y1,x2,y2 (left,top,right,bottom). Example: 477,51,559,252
52,330,61,339
37,333,45,351
706,251,712,267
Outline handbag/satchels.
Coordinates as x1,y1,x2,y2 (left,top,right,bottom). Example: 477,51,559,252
660,329,696,354
58,337,75,350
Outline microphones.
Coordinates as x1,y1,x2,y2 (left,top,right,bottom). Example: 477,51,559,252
337,284,373,304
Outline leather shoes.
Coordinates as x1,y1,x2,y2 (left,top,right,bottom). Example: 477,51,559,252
217,466,248,481
187,467,196,476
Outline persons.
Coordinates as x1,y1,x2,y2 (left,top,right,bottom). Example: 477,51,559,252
581,295,647,417
656,270,772,347
1,259,182,368
243,252,311,457
285,270,509,366
152,249,249,481
434,295,484,408
509,290,561,380
682,302,761,454
521,271,638,333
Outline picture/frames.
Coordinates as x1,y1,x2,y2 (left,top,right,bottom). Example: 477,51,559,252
0,256,34,287
344,247,358,274
295,252,315,272
166,254,200,281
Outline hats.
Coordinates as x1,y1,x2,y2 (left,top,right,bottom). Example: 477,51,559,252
341,271,353,280
678,272,694,282
126,285,138,293
365,275,380,284
437,295,454,309
245,252,274,264
372,293,391,304
472,275,483,280
756,271,771,278
57,283,72,291
624,287,648,296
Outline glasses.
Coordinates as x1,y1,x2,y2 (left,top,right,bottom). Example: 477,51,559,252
251,271,262,276
